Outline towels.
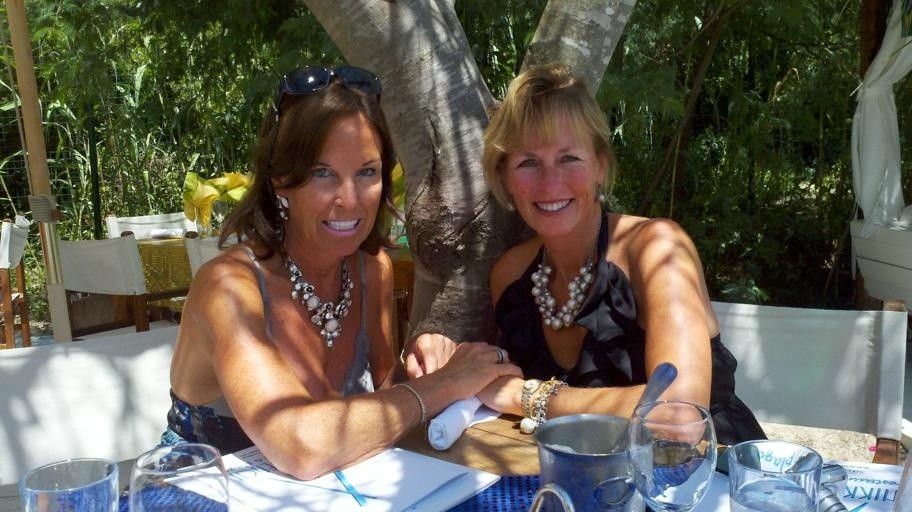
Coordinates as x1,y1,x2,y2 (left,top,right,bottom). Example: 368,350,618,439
428,396,502,451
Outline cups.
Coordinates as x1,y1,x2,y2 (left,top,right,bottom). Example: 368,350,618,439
22,459,119,512
730,440,823,511
196,219,212,239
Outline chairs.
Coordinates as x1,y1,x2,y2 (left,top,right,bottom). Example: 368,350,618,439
55,232,178,341
185,231,247,280
711,301,909,463
0,329,176,511
105,213,199,330
0,212,33,348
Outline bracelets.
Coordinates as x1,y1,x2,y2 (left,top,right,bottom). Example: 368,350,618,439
532,376,568,424
392,382,426,435
400,348,405,365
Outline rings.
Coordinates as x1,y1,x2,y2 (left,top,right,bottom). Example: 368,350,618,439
495,347,504,365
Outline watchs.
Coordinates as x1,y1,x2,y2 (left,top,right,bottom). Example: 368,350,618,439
520,378,543,418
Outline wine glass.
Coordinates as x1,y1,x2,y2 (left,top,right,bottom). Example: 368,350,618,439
212,200,229,236
630,401,718,512
130,443,229,512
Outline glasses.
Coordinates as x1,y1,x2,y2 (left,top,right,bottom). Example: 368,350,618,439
277,66,382,107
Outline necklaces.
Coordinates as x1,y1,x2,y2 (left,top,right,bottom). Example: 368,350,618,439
529,239,597,331
278,249,356,352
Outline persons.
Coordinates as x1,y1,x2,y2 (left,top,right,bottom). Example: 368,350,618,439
476,61,768,449
162,77,527,480
